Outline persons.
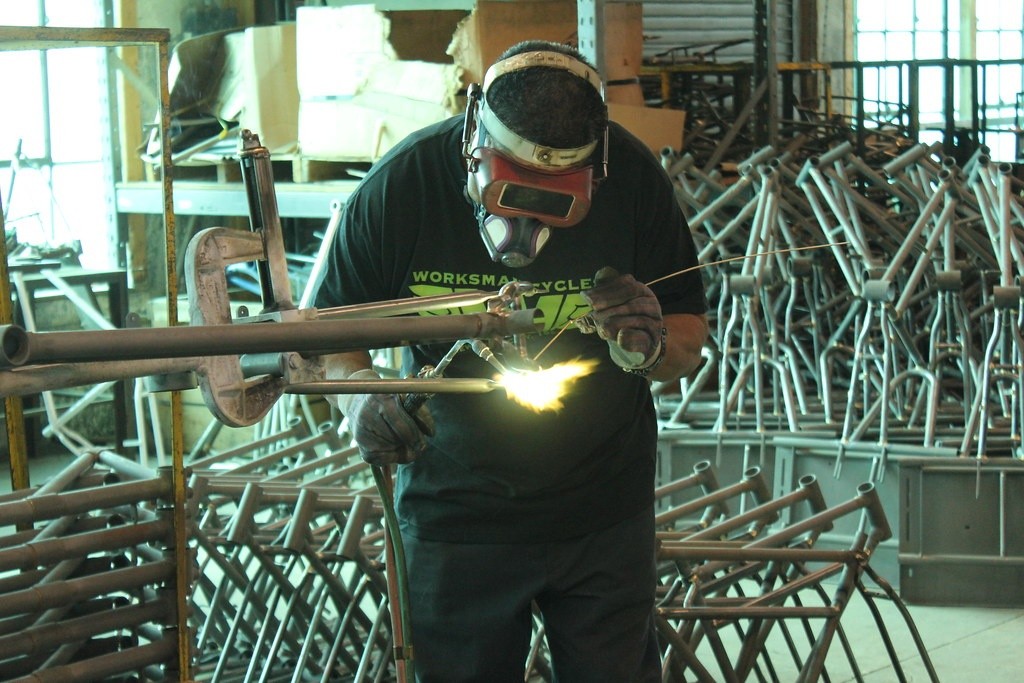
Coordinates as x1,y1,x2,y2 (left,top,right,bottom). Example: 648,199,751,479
296,39,712,683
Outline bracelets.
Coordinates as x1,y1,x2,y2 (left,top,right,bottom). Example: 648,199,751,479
622,321,668,377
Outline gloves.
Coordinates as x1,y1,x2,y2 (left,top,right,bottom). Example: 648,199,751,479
573,264,668,377
336,369,437,467
